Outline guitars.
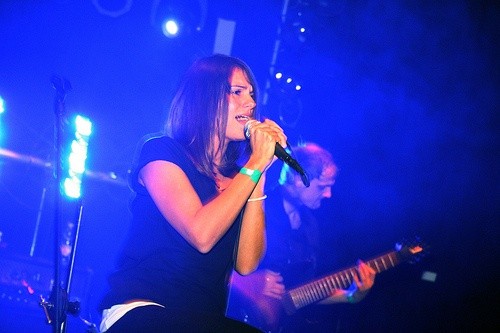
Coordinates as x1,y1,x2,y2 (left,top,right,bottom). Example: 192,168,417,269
246,241,429,333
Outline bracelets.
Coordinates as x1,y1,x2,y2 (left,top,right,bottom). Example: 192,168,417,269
247,193,268,202
348,288,356,305
240,166,261,183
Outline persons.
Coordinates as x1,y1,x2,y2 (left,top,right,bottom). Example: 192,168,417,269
101,50,289,333
225,141,377,332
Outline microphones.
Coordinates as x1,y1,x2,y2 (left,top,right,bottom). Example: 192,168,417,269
245,119,305,174
